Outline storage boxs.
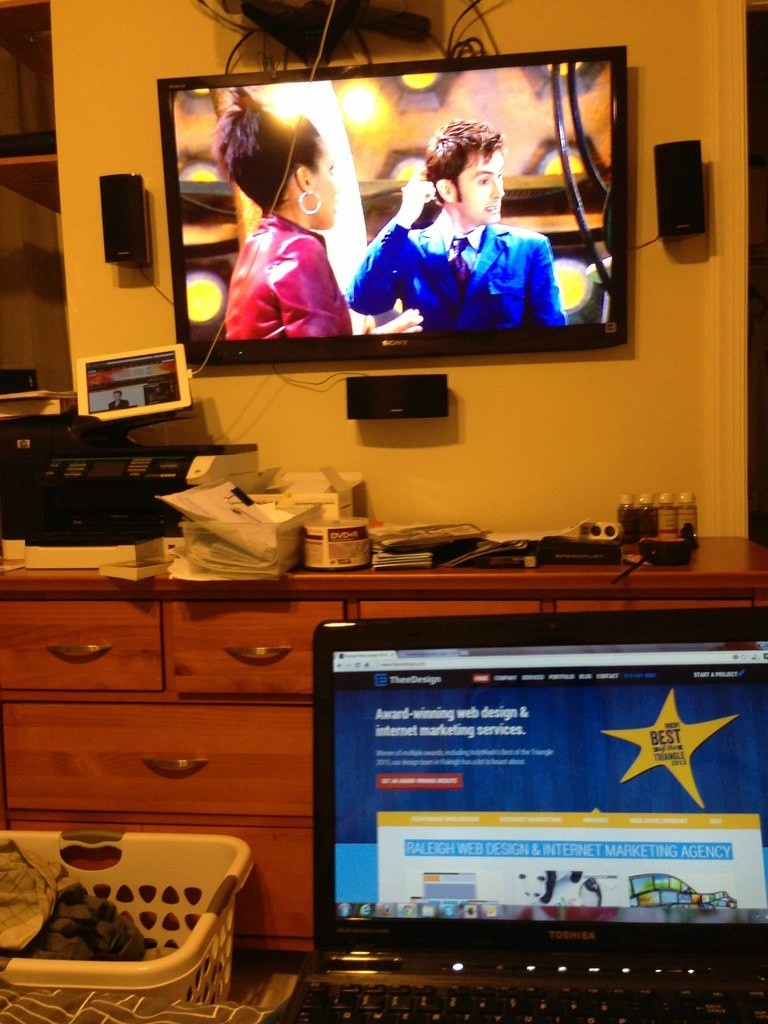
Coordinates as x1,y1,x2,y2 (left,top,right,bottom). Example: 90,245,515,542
179,501,324,575
0,830,254,1024
246,466,367,519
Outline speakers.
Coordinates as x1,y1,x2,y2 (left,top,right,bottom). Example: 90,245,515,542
656,140,705,237
99,174,150,263
346,374,450,420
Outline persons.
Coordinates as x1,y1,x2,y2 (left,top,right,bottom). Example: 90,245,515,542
214,85,422,341
109,391,130,410
344,117,565,333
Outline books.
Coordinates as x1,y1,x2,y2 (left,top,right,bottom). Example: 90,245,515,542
0,391,77,419
99,560,171,582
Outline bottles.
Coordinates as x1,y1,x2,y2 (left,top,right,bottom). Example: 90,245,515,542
657,493,677,541
636,493,657,541
677,492,698,541
617,494,636,545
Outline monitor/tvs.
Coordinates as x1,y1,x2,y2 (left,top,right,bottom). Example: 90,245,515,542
158,46,628,364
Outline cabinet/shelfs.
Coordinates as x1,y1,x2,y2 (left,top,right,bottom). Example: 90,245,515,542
0,537,768,951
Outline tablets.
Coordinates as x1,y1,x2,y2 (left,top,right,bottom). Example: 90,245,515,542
76,344,192,423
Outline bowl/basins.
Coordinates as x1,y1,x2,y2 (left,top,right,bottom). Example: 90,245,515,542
639,537,700,565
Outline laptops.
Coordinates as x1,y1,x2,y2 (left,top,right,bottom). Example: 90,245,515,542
282,607,768,1024
